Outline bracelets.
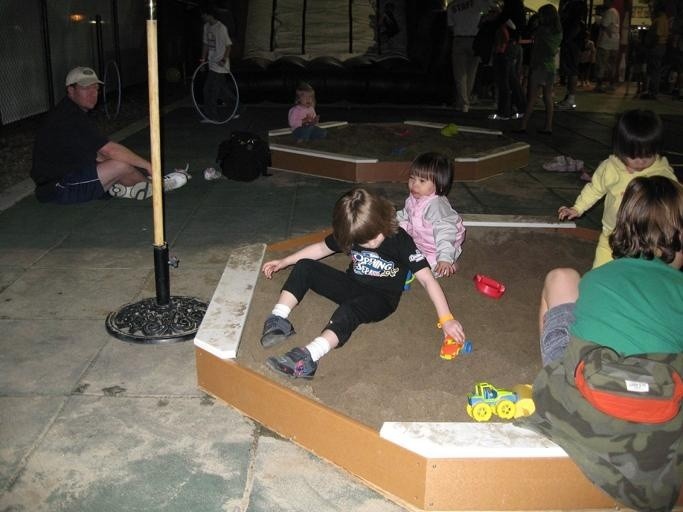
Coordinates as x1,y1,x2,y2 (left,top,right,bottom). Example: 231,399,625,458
436,314,454,328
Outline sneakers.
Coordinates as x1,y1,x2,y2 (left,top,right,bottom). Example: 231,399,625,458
147,172,188,192
202,167,222,181
264,346,319,381
535,129,552,139
232,114,241,120
260,314,296,348
624,82,683,104
108,181,153,201
511,128,529,139
511,112,525,119
554,79,616,94
174,163,192,180
486,113,510,121
542,155,592,183
199,118,218,124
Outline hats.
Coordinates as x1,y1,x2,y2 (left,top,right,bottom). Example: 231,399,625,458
295,78,313,97
65,66,105,87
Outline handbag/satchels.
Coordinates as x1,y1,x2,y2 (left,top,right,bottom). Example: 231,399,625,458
217,130,273,182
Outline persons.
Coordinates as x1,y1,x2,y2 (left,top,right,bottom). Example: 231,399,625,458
557,110,683,281
27,65,187,203
197,6,240,124
533,173,683,507
371,1,682,138
256,188,465,384
286,82,327,146
390,150,467,283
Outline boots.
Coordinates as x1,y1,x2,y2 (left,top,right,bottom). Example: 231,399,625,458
553,94,577,111
